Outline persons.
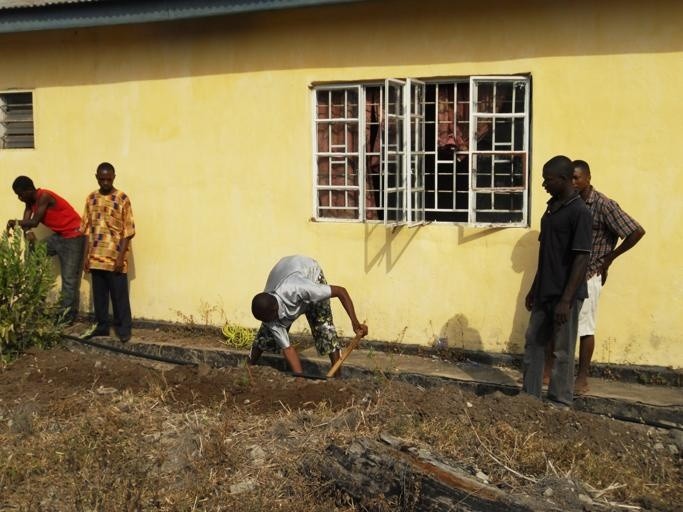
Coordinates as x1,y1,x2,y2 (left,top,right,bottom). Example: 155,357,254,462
78,161,137,343
6,175,86,320
248,253,369,383
520,154,595,411
516,158,647,397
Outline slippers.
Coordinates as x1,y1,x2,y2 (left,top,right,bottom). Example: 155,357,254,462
518,374,589,396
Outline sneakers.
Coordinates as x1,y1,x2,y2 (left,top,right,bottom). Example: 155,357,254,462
119,333,131,343
81,330,109,337
